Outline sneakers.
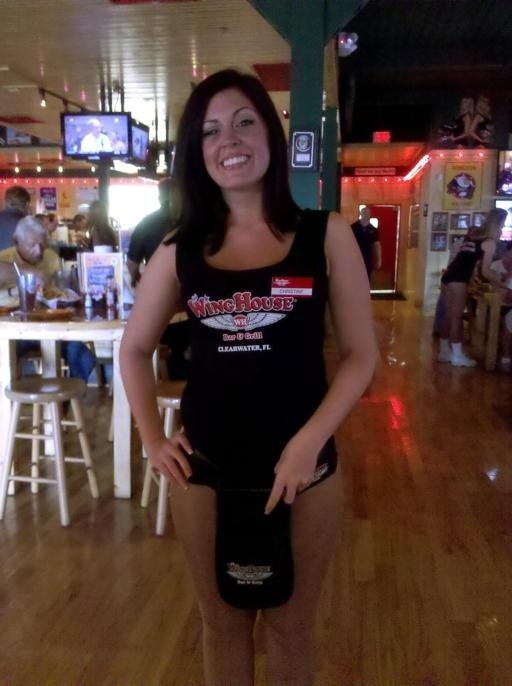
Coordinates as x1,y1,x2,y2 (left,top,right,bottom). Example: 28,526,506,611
451,354,476,368
437,351,453,364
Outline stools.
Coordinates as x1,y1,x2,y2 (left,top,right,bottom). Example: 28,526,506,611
0,375,103,528
139,378,190,537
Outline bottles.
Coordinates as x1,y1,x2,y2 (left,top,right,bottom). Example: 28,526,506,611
106,287,115,305
107,306,116,321
85,307,94,321
84,291,93,307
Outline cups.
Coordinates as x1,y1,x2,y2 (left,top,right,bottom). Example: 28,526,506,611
16,272,37,315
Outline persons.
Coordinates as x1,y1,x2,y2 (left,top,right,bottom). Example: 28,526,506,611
127,181,189,382
436,206,511,368
348,205,383,279
119,70,382,685
66,116,125,153
1,186,117,387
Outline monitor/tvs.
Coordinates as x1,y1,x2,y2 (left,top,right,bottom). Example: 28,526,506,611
122,118,149,166
495,148,512,196
60,112,131,159
494,199,512,241
